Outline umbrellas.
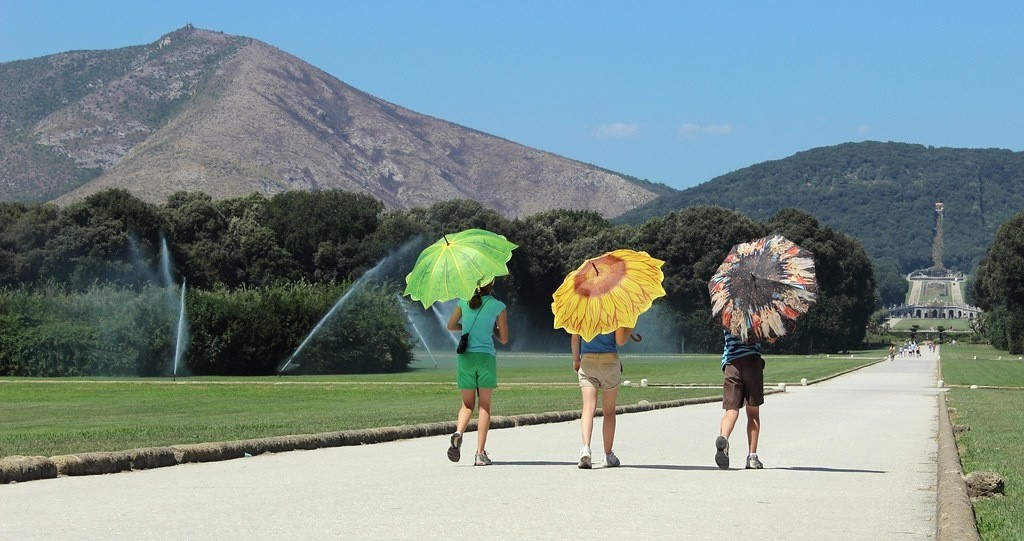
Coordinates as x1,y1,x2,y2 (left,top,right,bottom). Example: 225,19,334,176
551,249,666,342
402,228,519,309
708,234,817,345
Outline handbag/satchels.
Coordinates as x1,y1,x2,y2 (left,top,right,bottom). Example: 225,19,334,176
457,333,468,353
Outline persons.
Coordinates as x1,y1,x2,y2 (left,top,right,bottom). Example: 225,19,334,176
715,328,777,468
572,327,632,469
448,278,507,465
889,340,937,362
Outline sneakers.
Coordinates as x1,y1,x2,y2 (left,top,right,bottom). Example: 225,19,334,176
715,436,729,470
578,447,592,469
746,456,763,469
474,451,491,466
602,452,620,468
448,431,462,462
